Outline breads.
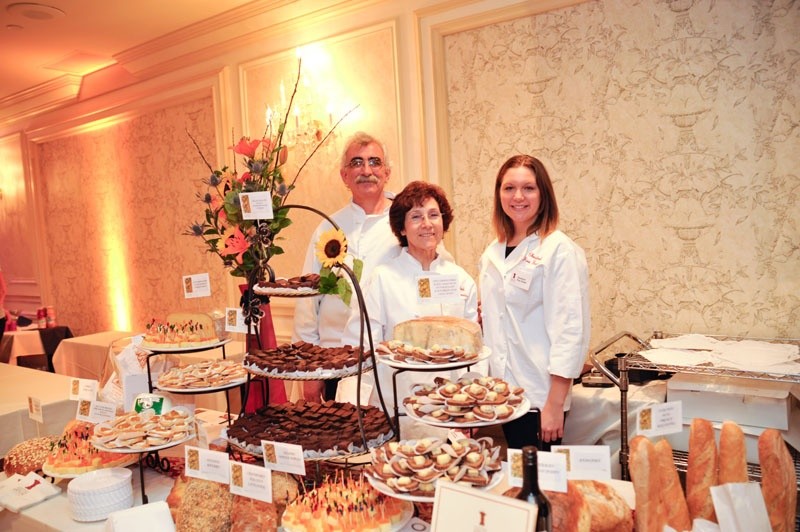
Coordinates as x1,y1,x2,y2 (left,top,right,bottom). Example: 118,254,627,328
499,417,797,532
60,418,96,438
140,319,218,348
2,436,56,478
394,315,483,355
164,309,230,341
167,469,298,532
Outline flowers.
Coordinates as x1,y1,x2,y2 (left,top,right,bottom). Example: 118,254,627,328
185,56,360,305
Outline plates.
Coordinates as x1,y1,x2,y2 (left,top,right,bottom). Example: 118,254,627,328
155,372,256,392
376,340,492,369
67,468,135,522
90,432,195,452
133,333,232,351
365,462,504,501
42,453,146,478
403,395,531,427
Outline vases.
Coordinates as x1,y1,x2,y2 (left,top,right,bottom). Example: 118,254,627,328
237,284,288,414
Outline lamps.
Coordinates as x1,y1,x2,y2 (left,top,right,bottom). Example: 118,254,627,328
263,75,337,146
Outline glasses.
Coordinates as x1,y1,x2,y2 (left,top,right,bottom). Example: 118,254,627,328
344,158,386,169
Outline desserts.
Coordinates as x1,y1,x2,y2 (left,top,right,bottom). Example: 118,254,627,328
366,438,503,494
405,376,523,423
222,400,393,458
243,340,374,378
254,273,321,293
282,478,409,532
91,408,194,449
45,432,133,476
157,357,246,389
376,339,479,363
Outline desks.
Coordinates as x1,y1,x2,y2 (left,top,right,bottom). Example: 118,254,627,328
0,326,74,372
52,330,134,380
0,363,94,455
0,406,238,532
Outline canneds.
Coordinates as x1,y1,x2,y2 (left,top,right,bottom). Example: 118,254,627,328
36,306,56,329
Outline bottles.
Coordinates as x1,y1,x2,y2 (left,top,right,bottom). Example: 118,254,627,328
515,446,553,532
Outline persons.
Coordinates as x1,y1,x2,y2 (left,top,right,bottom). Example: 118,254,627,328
481,153,590,452
294,133,403,404
343,182,482,436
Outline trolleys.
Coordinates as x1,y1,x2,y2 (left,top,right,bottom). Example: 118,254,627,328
588,329,800,532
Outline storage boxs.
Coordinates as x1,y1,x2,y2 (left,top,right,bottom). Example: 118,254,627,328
663,373,792,466
